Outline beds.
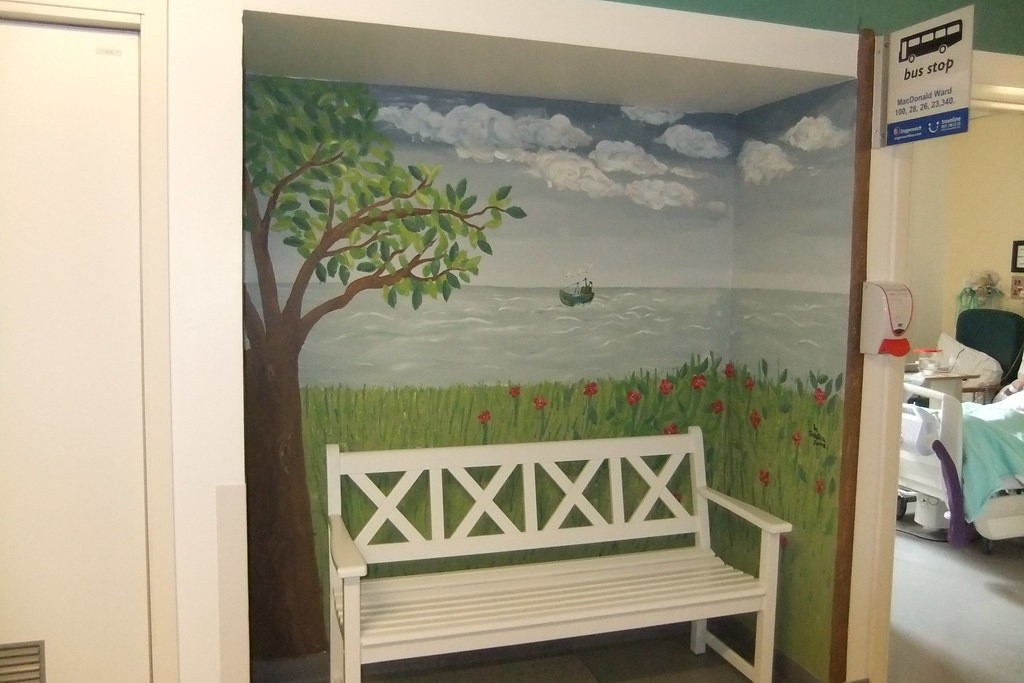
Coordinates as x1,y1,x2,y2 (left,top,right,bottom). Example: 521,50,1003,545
896,341,1024,549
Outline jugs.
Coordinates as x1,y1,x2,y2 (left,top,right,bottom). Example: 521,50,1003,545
912,348,942,377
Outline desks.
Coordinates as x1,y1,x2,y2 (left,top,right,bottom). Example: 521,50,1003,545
919,372,980,410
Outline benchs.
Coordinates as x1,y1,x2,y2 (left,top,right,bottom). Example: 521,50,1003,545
324,427,793,683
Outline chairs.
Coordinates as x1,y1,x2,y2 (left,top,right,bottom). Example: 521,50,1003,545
919,331,1003,403
905,308,1023,404
